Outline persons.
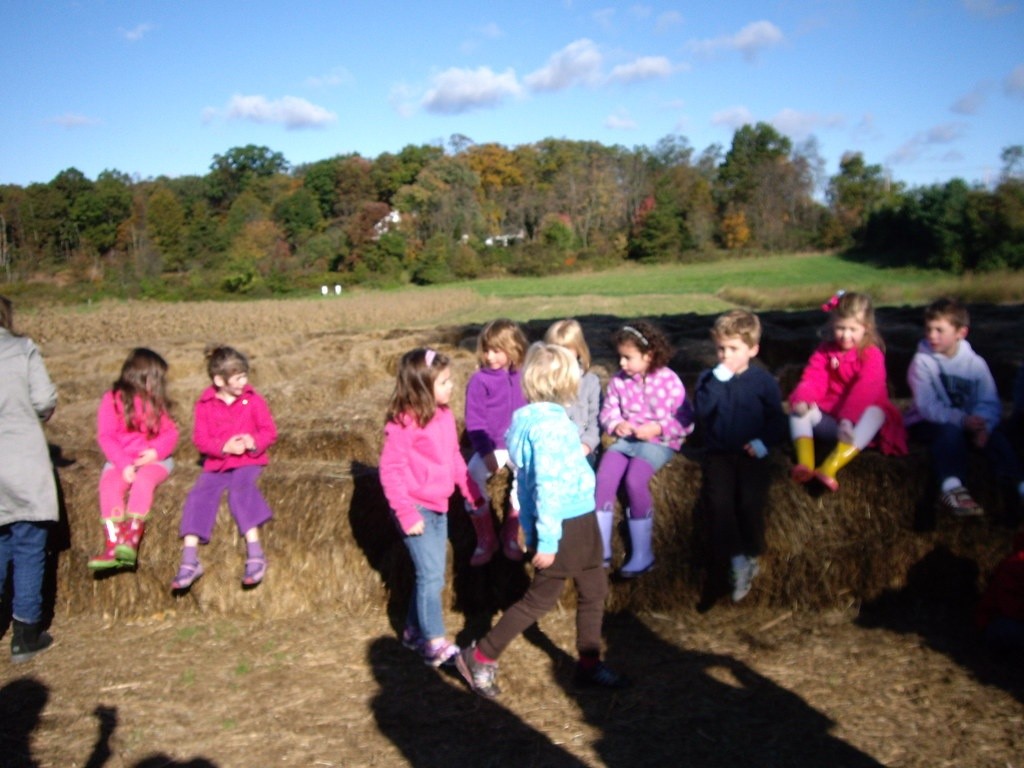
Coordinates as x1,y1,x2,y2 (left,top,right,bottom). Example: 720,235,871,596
1007,362,1024,495
783,291,908,489
686,310,789,602
464,317,531,567
594,320,693,578
456,340,631,698
0,293,59,663
544,318,602,472
378,349,486,669
910,298,1005,516
171,341,277,589
86,346,178,569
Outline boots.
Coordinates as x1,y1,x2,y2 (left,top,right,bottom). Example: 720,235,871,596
114,512,146,567
815,441,860,492
619,518,657,578
790,436,816,484
86,516,125,569
595,511,614,568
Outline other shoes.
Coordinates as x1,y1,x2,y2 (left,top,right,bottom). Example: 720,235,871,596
241,552,269,584
941,486,984,517
575,660,631,694
503,507,524,560
470,509,498,565
8,620,55,662
454,641,504,700
726,549,761,603
422,640,460,667
401,625,426,649
172,559,203,588
